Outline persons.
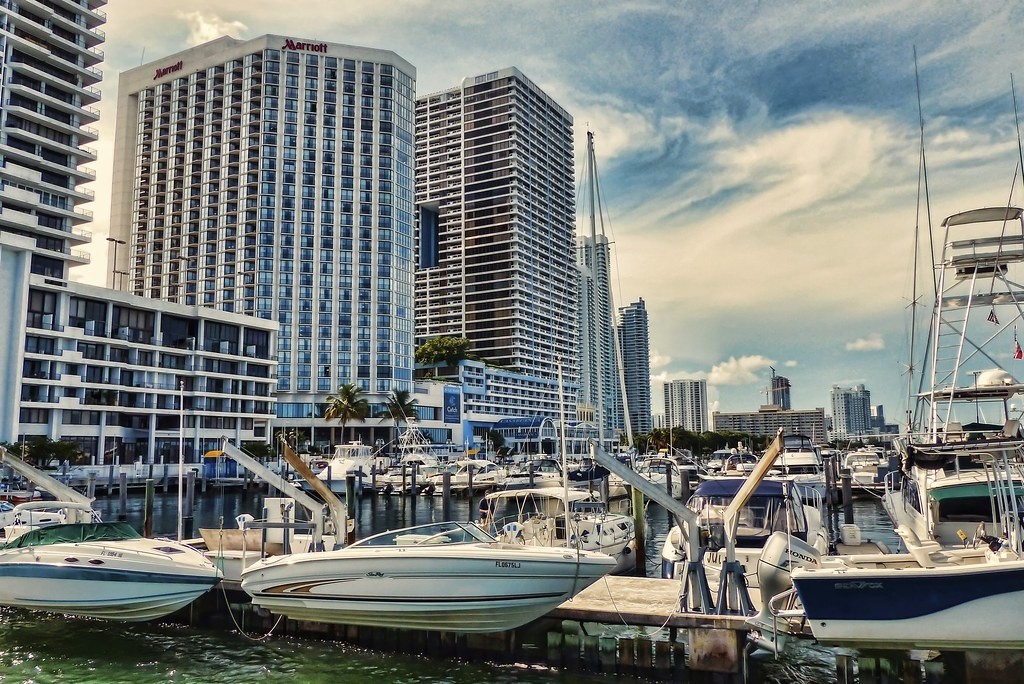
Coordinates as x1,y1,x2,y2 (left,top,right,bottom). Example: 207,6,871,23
478,489,496,534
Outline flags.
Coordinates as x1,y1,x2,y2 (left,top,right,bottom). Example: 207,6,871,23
986,310,1001,326
1012,341,1022,360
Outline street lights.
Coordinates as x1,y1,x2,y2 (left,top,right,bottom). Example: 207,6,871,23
106,238,126,290
112,269,130,291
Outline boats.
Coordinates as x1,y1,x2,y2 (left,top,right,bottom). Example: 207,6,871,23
238,520,621,633
0,42,1024,653
